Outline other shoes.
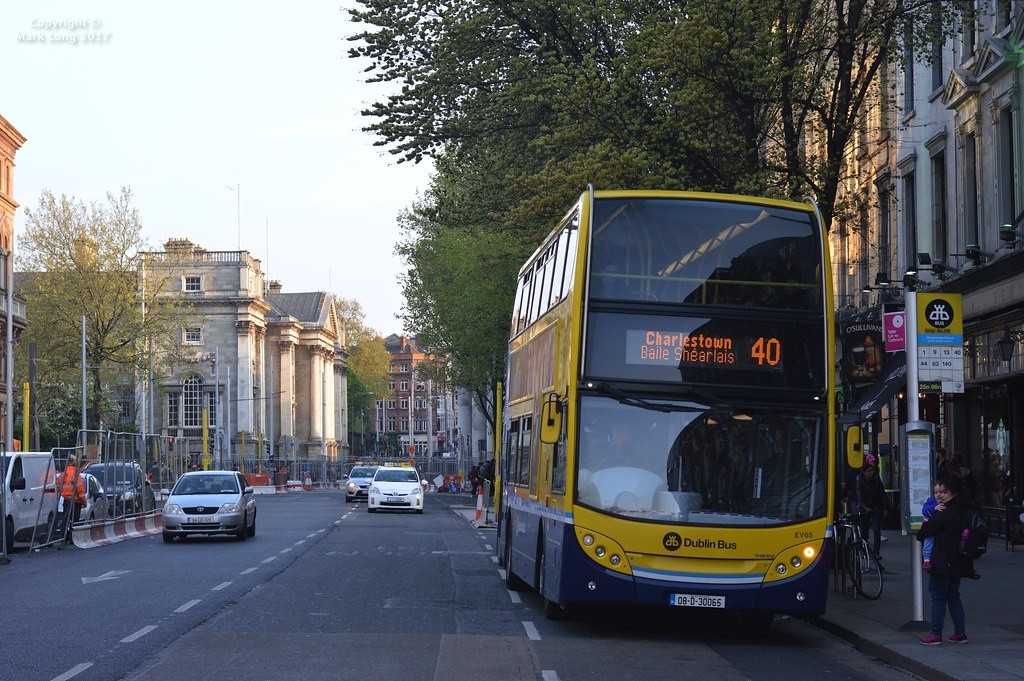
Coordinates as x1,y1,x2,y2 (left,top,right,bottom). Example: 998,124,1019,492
881,536,888,542
948,634,968,643
923,561,932,569
920,635,943,645
971,574,981,579
874,553,882,560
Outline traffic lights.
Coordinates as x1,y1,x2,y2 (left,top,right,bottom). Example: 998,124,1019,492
170,438,173,451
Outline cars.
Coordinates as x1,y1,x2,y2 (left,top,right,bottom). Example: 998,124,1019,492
160,470,257,543
56,473,110,532
343,466,379,503
364,466,428,514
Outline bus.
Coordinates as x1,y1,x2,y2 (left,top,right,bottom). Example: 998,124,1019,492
493,182,863,632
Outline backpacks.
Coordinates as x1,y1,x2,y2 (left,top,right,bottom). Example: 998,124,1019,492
480,463,491,478
952,511,988,560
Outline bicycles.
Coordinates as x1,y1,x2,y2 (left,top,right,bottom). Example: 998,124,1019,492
833,512,883,600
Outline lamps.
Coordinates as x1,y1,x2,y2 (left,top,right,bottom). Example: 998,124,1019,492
862,286,904,293
999,224,1024,241
966,245,994,259
996,324,1024,361
875,272,903,285
903,253,959,286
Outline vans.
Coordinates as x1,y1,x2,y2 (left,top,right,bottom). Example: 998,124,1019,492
0,451,58,555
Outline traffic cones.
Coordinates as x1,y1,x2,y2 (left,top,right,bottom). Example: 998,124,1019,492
475,485,483,521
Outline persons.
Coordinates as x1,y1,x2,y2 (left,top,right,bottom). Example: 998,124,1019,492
56,454,86,523
397,436,403,457
590,261,631,298
607,422,640,464
185,477,200,492
410,472,417,480
187,463,204,472
151,461,174,489
916,448,981,645
423,445,428,457
231,463,239,471
844,463,888,560
982,448,1011,506
409,444,414,457
468,459,496,497
383,433,389,457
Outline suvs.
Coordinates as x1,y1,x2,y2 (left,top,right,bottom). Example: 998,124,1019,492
80,461,156,522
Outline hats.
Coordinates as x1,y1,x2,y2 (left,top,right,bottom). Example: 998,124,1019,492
67,453,76,464
866,455,875,463
